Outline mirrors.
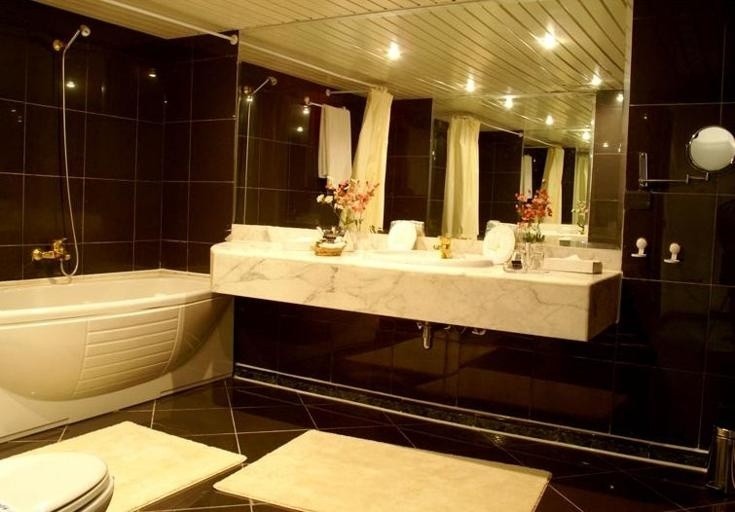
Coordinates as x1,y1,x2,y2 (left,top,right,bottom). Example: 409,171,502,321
231,0,633,251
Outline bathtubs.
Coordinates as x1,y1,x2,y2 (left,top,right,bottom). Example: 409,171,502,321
486,219,588,248
0,267,235,447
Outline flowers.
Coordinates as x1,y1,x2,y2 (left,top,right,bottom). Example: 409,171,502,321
316,179,363,230
353,180,380,214
512,190,536,243
533,186,552,243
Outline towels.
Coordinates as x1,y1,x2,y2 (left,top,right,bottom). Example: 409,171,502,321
317,103,353,190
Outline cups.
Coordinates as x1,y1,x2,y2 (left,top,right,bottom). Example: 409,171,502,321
511,250,522,270
577,238,596,262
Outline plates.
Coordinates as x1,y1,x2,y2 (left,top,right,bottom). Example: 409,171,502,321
384,221,417,252
481,225,515,265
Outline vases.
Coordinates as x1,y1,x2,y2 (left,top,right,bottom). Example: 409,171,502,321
529,230,546,271
340,224,358,252
354,213,368,239
503,230,528,273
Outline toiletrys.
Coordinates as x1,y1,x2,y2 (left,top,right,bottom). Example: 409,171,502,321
315,230,346,256
511,252,522,270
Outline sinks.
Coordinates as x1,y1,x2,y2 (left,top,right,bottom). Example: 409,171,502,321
341,249,493,267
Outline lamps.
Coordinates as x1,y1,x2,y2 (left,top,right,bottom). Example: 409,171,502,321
637,125,735,191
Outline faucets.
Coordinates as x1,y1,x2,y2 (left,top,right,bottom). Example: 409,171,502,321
432,234,454,260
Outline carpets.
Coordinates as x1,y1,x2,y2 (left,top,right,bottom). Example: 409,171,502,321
0,416,247,512
214,427,552,511
